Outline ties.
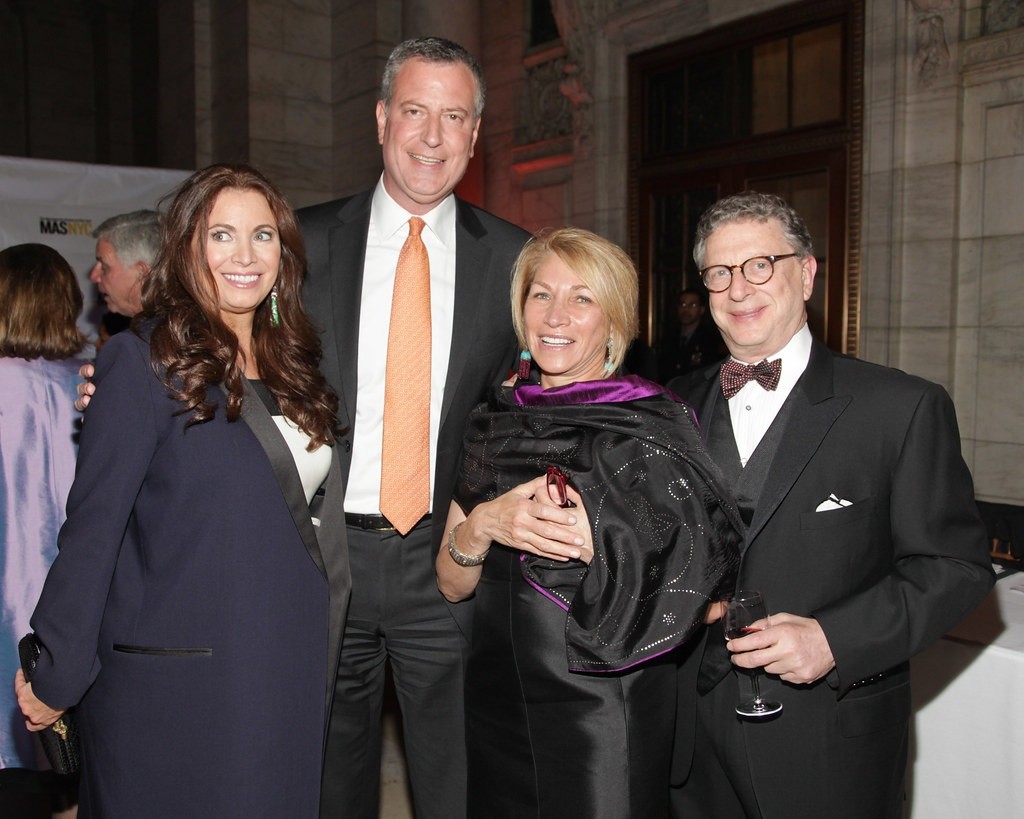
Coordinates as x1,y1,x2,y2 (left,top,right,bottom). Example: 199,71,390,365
379,217,430,537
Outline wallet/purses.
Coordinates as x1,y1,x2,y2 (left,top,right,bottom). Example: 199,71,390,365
18,635,80,776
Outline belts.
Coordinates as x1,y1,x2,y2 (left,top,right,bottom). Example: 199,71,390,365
342,513,431,531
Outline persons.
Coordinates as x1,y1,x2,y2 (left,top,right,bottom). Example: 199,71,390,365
435,225,742,819
15,164,352,819
90,210,164,318
0,243,85,819
657,289,732,377
619,192,998,819
75,39,537,819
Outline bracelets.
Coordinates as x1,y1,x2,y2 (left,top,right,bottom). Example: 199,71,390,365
448,521,490,567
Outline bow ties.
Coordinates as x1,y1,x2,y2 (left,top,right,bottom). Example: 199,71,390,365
719,358,782,401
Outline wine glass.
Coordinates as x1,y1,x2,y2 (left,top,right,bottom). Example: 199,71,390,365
721,588,783,716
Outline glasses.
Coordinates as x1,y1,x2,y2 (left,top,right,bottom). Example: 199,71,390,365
545,464,571,509
678,302,700,309
700,252,801,294
